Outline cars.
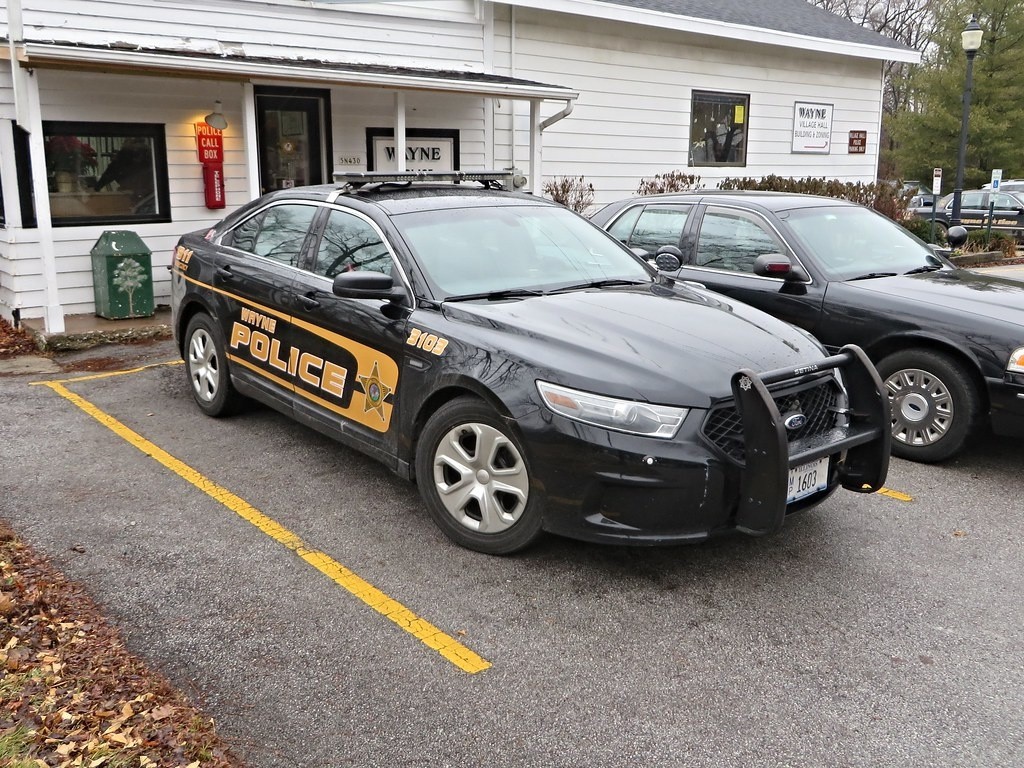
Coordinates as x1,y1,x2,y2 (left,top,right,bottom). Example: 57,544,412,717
588,191,1024,463
172,174,892,558
895,180,1023,247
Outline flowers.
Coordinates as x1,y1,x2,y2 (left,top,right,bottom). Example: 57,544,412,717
45,136,99,177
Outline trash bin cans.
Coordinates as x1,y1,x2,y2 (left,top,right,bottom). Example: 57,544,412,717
89,230,155,321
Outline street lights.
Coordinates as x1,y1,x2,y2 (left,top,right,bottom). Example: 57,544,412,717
949,13,984,225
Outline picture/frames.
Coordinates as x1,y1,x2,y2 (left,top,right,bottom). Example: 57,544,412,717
280,111,304,137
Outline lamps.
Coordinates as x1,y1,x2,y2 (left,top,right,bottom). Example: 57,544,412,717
205,100,228,130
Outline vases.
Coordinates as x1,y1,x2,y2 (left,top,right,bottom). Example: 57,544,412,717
55,168,80,193
